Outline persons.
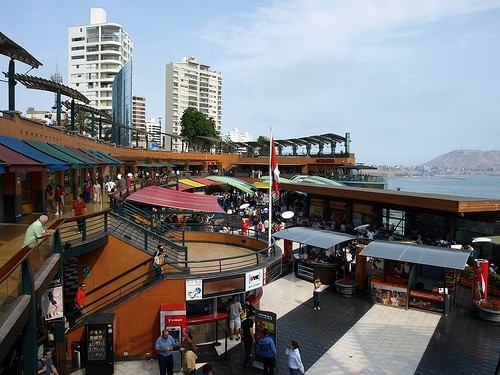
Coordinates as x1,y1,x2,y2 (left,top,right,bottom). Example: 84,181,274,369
220,190,289,234
239,310,257,367
312,277,322,311
155,329,175,375
45,184,87,233
73,283,88,316
81,166,177,204
47,290,61,312
163,213,215,232
259,327,276,375
38,348,59,375
153,244,166,278
228,295,255,341
24,215,56,246
290,210,475,265
285,340,304,375
179,331,214,375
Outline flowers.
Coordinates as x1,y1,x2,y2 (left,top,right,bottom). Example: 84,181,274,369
342,276,350,285
481,299,500,310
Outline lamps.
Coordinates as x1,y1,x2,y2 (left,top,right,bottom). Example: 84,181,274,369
63,243,71,253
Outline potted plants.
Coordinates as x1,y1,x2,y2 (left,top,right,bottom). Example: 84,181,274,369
461,267,473,287
487,272,500,296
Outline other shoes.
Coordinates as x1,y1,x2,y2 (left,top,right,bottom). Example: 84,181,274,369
54,213,59,216
314,307,316,311
229,336,233,340
60,211,62,214
318,306,320,310
236,336,240,341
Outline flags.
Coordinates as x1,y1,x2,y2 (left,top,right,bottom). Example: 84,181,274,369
272,138,281,196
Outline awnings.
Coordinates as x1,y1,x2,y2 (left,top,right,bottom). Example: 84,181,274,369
0,136,125,175
133,161,270,195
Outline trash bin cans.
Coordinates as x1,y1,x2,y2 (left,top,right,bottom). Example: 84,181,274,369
71,342,82,368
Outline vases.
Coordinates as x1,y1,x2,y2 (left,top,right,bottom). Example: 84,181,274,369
478,305,500,322
335,279,359,297
